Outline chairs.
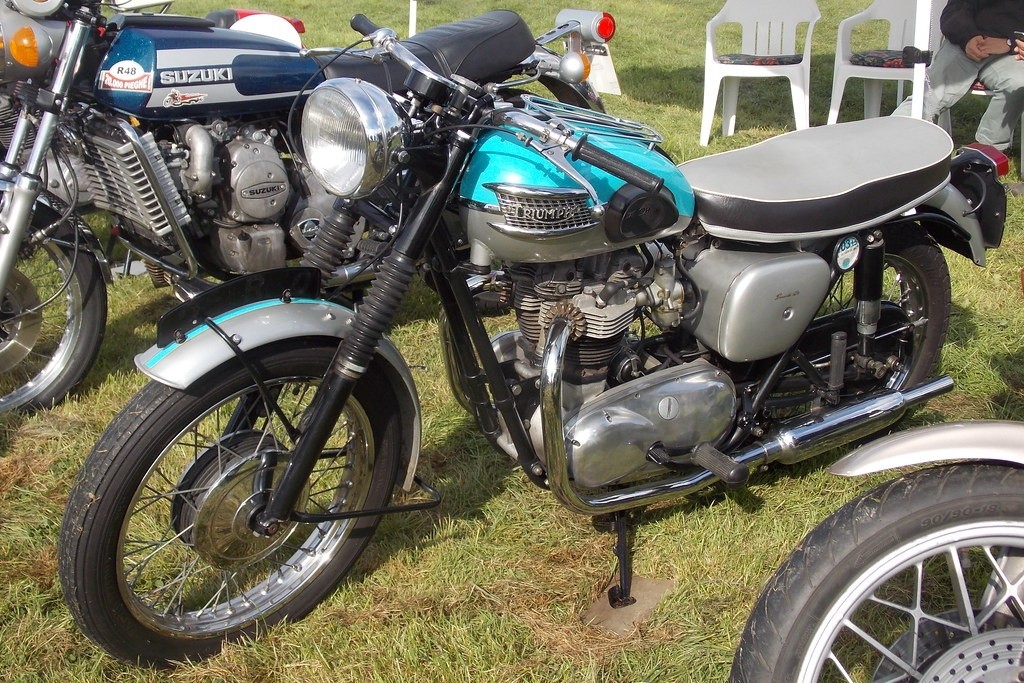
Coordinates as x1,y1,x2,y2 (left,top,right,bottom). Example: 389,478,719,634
699,1,822,148
827,0,948,125
932,31,1024,180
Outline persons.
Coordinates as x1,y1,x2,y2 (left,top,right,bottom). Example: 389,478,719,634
890,0,1024,157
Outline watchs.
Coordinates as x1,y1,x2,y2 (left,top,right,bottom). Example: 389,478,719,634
1006,38,1012,46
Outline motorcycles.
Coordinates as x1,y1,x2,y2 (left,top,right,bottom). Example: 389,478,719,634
0,1,623,429
54,15,1016,675
726,416,1024,683
